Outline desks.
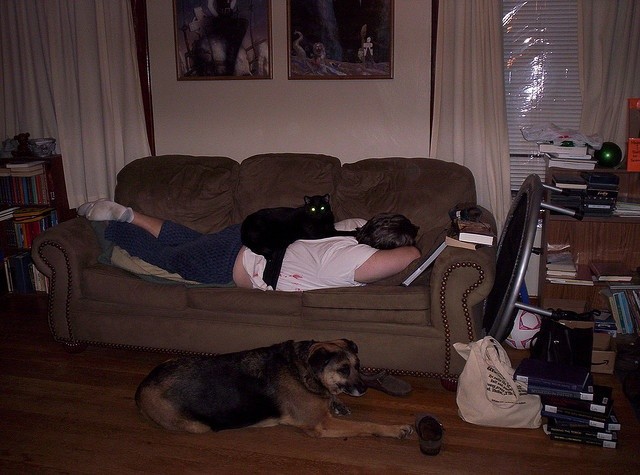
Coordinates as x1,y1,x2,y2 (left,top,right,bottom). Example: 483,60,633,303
484,173,583,354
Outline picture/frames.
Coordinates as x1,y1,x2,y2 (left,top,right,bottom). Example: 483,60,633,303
172,0,275,81
287,1,395,80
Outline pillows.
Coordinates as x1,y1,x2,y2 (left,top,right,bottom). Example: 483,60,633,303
379,221,453,285
125,162,242,238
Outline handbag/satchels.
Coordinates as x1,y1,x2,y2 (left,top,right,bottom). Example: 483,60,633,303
454,336,545,432
528,308,602,385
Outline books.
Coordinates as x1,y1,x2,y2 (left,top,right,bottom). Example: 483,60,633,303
589,260,633,281
527,373,594,402
0,174,67,206
545,158,595,171
606,275,639,289
12,169,46,177
0,210,61,245
512,357,590,391
395,225,448,287
1,167,12,177
553,170,640,218
544,151,592,160
10,163,44,173
545,153,598,164
540,385,613,415
593,287,640,341
445,220,478,251
4,249,50,291
548,433,620,450
6,159,46,168
540,407,621,431
546,265,594,286
451,215,493,246
542,402,612,422
538,141,587,155
546,252,577,271
547,423,618,442
546,262,578,277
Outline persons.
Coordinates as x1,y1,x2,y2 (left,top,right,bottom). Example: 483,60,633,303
76,198,423,290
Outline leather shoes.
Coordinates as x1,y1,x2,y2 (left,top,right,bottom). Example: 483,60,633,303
361,369,413,394
413,412,444,455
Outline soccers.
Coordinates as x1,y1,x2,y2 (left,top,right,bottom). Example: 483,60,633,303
505,308,542,350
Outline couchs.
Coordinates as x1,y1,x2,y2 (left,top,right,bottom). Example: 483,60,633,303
28,154,499,392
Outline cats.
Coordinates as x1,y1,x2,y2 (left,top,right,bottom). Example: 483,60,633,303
241,193,337,263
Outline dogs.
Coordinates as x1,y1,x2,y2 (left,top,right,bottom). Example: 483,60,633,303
135,337,413,442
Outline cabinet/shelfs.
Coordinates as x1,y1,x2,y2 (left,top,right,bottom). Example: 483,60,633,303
541,161,639,357
1,155,69,298
